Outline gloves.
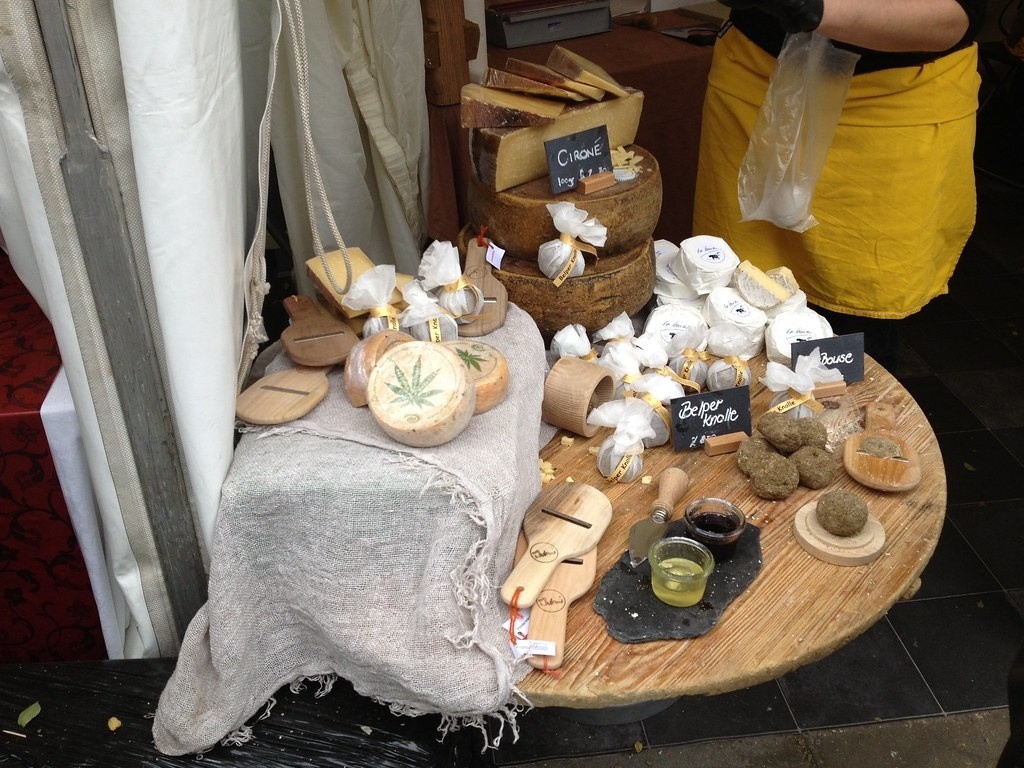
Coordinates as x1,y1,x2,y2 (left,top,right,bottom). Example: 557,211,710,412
721,0,823,32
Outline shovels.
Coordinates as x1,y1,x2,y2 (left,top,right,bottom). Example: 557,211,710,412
629,468,690,568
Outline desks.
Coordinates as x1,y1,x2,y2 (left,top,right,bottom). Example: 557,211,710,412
488,8,719,248
508,334,947,727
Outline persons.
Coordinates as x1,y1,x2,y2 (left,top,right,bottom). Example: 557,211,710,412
692,0,982,375
